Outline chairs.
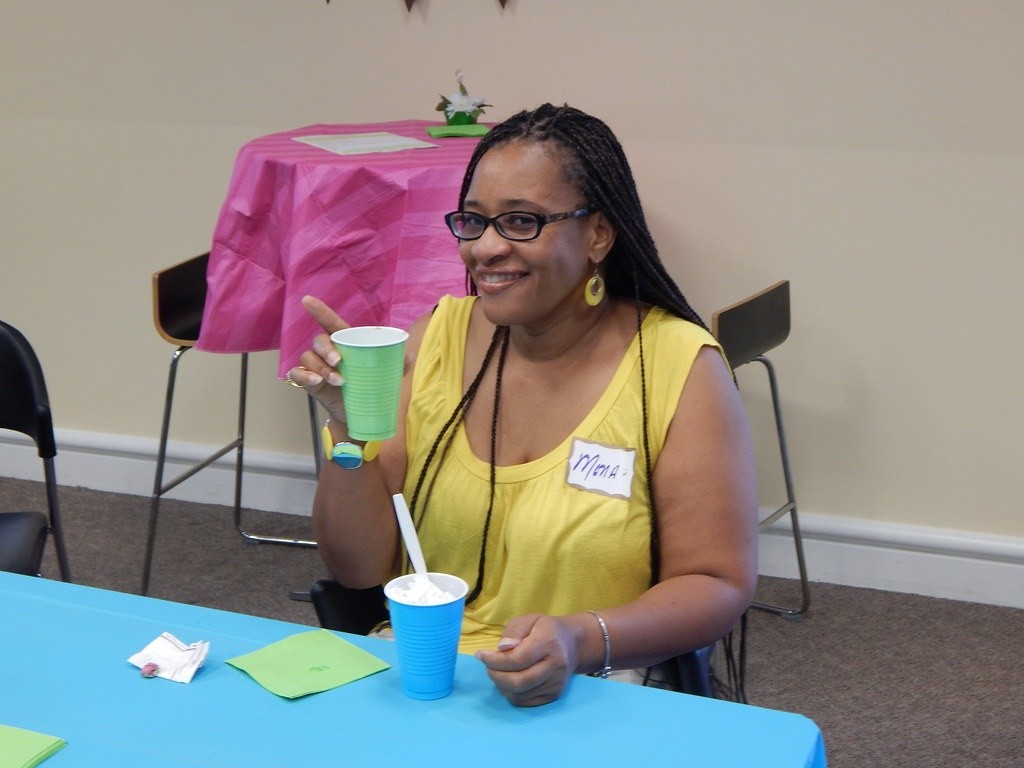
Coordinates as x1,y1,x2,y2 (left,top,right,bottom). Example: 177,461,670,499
0,322,72,583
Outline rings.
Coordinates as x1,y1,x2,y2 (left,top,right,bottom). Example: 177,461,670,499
285,366,308,388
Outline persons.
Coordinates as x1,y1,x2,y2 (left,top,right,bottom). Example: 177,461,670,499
289,103,759,709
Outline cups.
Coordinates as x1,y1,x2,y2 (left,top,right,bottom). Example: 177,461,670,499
330,326,406,440
383,573,469,701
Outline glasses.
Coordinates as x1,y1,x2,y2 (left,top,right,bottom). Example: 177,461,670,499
444,205,600,242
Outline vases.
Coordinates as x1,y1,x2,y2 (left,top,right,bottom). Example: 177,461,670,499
444,110,477,126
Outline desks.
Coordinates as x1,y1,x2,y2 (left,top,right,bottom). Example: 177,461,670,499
194,120,504,602
0,570,830,768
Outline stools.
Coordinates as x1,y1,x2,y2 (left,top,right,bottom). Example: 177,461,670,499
713,279,812,618
140,252,321,598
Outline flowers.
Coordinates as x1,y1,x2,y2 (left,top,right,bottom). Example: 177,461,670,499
435,81,494,119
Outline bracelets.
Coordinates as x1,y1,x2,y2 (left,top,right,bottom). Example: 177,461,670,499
321,419,384,469
587,612,612,680
